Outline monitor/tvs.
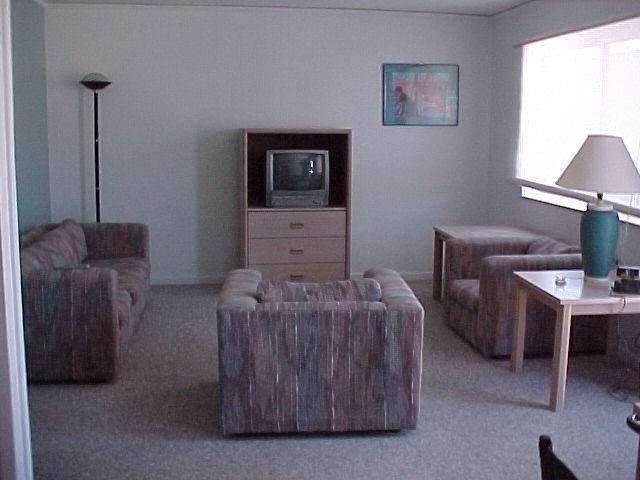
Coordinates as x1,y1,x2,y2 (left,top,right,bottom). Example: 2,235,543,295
265,149,330,208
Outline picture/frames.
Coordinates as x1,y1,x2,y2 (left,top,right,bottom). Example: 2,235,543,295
383,64,459,126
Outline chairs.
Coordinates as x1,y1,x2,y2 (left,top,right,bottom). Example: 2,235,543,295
443,238,608,359
216,267,425,436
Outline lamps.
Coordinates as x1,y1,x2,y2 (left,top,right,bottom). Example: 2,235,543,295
79,72,113,223
556,135,640,284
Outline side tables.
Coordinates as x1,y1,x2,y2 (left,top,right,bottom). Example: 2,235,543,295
432,225,541,300
509,270,640,412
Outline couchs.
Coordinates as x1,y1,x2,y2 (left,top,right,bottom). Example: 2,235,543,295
18,222,150,383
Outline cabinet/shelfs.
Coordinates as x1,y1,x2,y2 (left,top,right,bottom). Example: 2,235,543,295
240,128,351,282
